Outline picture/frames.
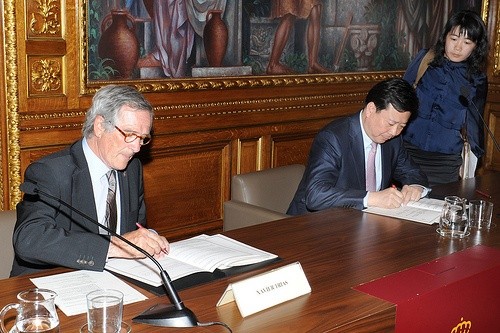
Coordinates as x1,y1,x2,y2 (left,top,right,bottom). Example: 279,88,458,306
77,0,490,96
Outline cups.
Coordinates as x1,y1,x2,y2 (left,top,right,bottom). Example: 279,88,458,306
469,199,494,232
86,288,124,333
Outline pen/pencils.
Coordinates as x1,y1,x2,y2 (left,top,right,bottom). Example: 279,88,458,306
135,222,170,255
476,189,491,199
391,184,404,205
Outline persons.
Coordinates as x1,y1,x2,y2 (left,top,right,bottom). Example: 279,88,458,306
285,76,433,217
403,9,491,193
7,83,171,279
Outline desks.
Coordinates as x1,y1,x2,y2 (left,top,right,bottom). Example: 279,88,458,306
1,171,500,333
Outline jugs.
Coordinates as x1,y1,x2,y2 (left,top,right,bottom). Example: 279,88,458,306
439,196,469,238
0,287,60,333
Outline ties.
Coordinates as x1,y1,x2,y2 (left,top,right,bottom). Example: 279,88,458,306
366,142,378,192
105,170,118,236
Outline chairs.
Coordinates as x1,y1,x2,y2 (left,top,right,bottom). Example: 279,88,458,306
224,164,306,232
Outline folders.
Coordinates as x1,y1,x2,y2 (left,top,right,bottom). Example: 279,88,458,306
103,232,283,298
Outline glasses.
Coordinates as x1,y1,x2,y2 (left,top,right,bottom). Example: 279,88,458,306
109,121,152,146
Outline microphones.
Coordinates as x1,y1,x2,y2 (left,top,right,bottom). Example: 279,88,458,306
19,182,199,327
459,84,500,152
